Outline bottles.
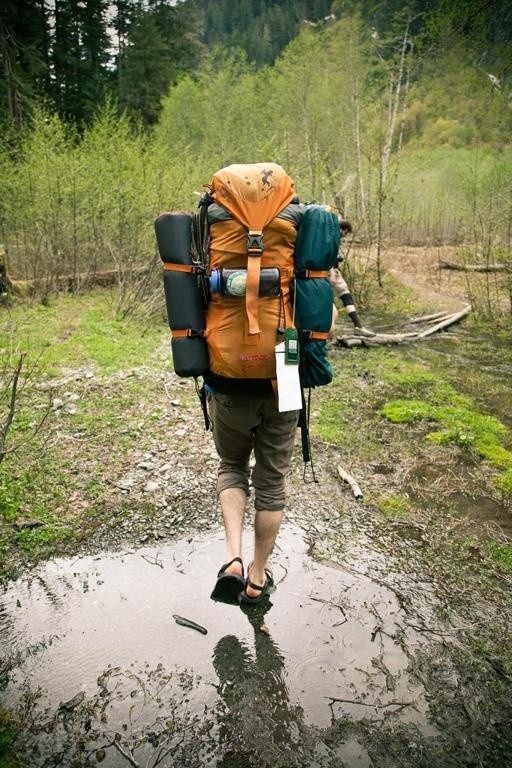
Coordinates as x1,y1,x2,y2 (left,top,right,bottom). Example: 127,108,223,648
286,328,299,364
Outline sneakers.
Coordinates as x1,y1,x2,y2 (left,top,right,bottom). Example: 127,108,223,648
353,326,376,337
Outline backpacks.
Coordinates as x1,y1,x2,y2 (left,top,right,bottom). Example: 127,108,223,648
153,163,340,388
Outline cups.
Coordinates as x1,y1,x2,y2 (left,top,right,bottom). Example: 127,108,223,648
209,267,281,299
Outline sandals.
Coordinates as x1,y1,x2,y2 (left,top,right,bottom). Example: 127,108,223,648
210,556,273,604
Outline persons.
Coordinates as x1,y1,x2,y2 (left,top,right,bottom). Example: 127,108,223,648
328,221,377,339
191,394,300,604
